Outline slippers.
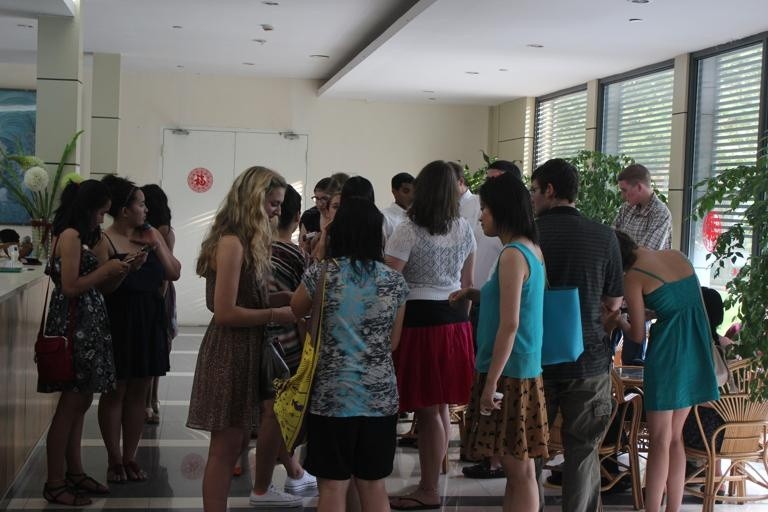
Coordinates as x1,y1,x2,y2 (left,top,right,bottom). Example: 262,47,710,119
389,495,442,511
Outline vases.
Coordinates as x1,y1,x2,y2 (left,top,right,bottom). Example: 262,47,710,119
31,219,51,262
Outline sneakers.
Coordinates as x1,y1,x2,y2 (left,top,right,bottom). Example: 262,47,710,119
283,466,316,494
248,485,303,508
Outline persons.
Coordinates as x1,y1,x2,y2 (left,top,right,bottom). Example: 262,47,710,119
186,161,731,512
0,228,33,266
37,174,182,507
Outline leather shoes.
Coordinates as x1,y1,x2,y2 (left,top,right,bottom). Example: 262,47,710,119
462,461,507,479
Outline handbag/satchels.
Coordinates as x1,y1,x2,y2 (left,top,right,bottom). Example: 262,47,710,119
34,331,76,394
541,279,585,369
271,262,327,452
258,335,291,400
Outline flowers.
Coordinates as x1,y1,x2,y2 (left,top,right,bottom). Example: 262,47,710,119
0,130,85,260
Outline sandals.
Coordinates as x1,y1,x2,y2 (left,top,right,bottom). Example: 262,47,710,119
106,460,127,485
42,481,92,506
122,456,145,482
63,470,111,497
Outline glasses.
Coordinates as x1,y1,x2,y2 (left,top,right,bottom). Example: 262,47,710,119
311,195,328,203
